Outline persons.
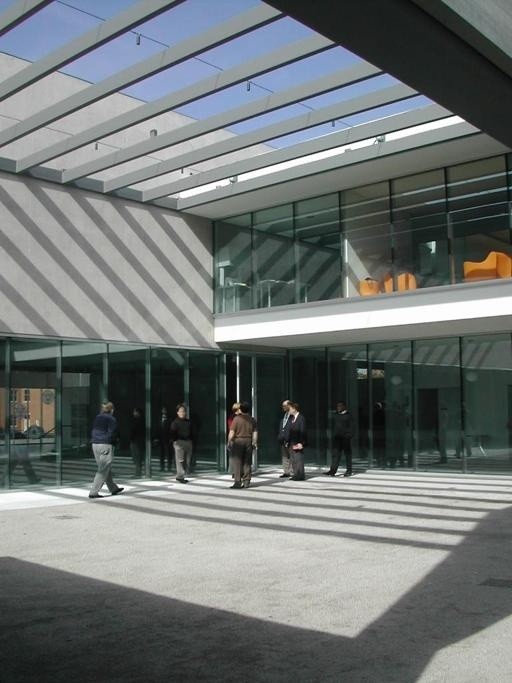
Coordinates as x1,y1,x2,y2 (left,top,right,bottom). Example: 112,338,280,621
227,402,243,436
321,401,357,476
158,406,173,474
453,400,473,459
434,407,450,465
225,400,259,488
284,401,306,481
276,398,294,478
2,414,43,486
128,406,148,476
170,403,195,483
363,397,420,471
88,401,125,498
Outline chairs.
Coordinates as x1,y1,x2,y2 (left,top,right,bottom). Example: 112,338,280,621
357,248,512,297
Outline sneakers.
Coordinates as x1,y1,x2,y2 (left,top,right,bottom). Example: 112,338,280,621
88,494,106,498
175,477,189,483
323,471,352,476
230,482,250,489
111,487,125,495
279,473,305,480
